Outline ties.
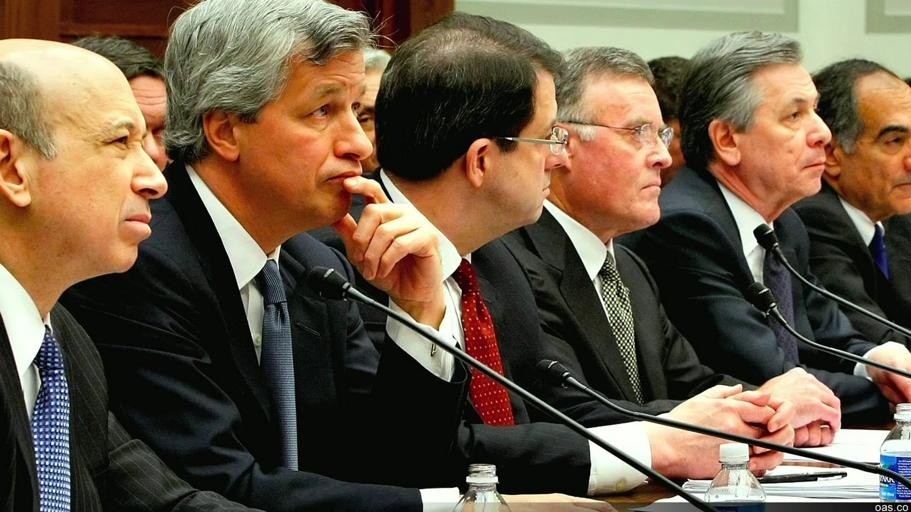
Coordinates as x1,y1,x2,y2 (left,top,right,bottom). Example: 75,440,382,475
254,260,299,472
30,329,72,512
764,247,799,365
452,259,516,426
600,252,645,406
870,223,891,282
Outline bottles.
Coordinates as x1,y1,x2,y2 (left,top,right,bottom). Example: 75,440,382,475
454,464,511,512
880,402,911,501
704,443,766,512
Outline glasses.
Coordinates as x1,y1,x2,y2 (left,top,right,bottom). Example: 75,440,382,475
504,127,570,154
566,116,675,148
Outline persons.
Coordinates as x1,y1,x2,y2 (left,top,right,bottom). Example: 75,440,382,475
0,1,911,512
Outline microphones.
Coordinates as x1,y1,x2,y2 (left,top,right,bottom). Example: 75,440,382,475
305,265,717,511
538,357,910,490
753,223,911,335
745,282,911,378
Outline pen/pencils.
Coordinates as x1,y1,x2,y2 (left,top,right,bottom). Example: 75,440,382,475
757,472,848,484
